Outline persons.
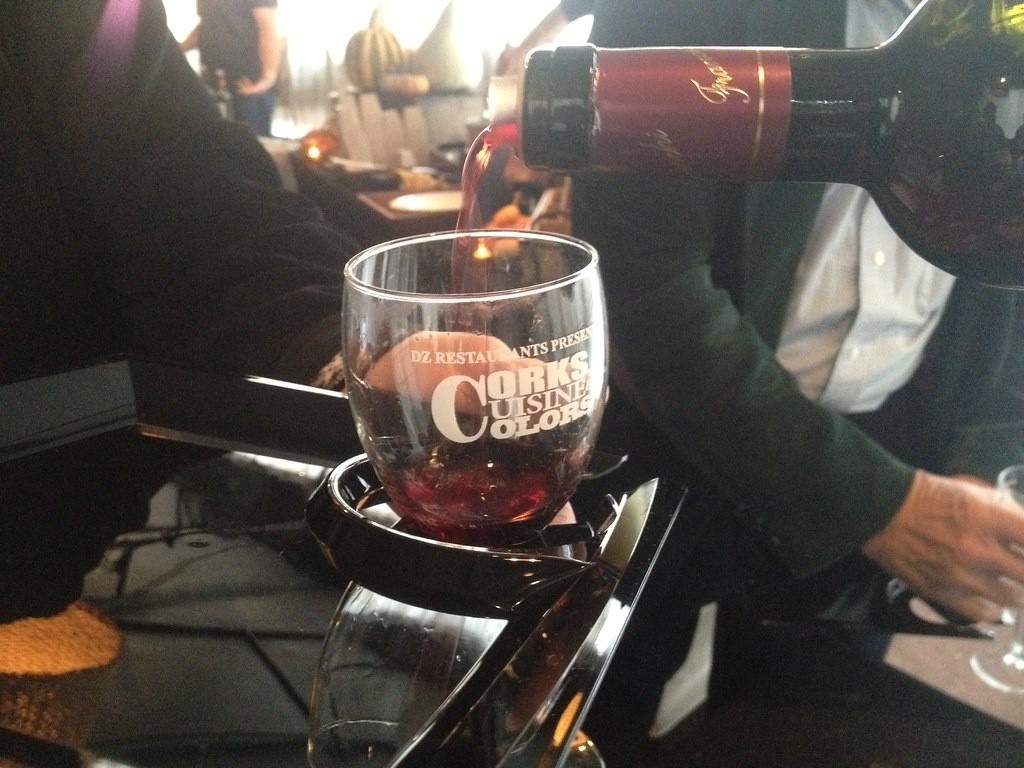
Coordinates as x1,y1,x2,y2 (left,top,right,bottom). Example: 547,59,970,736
496,0,592,75
180,0,277,136
569,1,1024,768
0,0,554,625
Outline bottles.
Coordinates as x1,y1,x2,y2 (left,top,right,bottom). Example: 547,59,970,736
523,0,1024,290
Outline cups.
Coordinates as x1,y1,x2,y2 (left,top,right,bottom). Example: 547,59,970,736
342,230,612,545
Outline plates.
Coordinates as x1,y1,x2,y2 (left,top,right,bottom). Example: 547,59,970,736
389,192,468,211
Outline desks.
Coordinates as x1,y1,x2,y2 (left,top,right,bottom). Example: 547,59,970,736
356,187,463,237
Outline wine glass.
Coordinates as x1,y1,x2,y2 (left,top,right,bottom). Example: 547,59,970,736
970,465,1024,694
